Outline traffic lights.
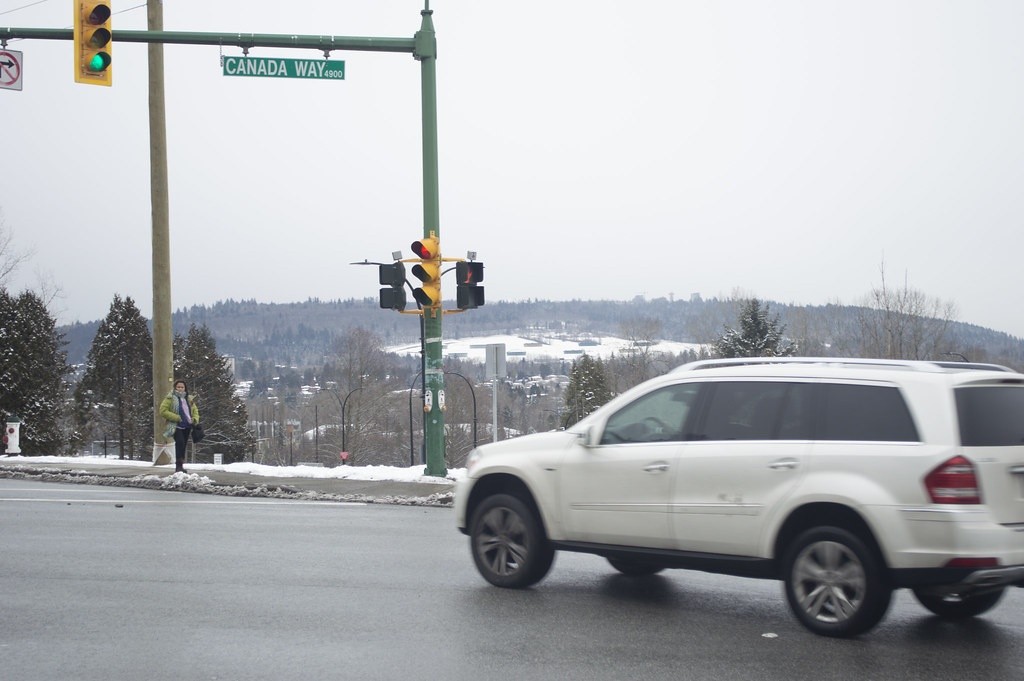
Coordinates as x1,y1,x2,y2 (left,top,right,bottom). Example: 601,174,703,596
457,261,485,310
410,236,442,308
73,0,113,86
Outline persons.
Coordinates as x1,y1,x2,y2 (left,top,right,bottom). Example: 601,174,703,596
160,379,199,474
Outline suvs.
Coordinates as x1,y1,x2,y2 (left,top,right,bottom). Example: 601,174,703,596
452,356,1024,639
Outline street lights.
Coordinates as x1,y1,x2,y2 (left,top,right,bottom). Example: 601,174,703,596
321,387,365,466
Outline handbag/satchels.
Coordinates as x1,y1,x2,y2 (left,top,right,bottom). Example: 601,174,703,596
191,423,205,443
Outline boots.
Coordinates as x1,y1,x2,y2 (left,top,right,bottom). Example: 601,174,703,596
176,457,187,473
